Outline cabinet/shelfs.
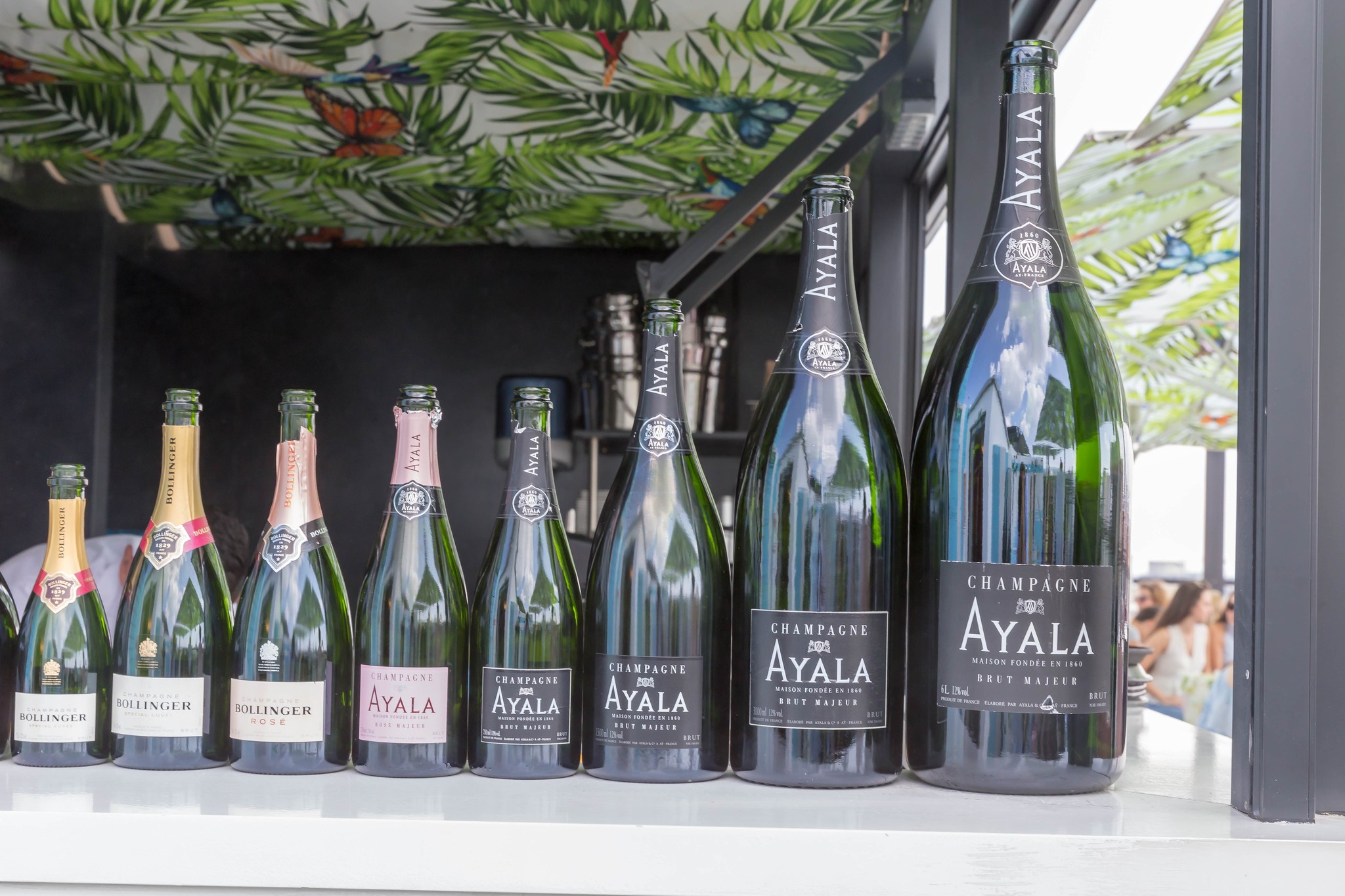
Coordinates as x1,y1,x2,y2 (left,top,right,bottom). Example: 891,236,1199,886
566,431,749,567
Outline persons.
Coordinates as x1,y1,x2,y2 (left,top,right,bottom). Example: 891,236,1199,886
1128,580,1214,722
1136,580,1167,610
1205,588,1234,675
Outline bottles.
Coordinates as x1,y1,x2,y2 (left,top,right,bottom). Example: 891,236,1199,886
354,384,472,778
10,462,113,769
582,298,731,785
107,387,235,771
228,388,355,776
0,570,21,755
468,386,586,780
721,172,908,789
906,40,1132,798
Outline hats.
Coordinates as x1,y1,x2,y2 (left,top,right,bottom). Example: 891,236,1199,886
203,514,249,605
